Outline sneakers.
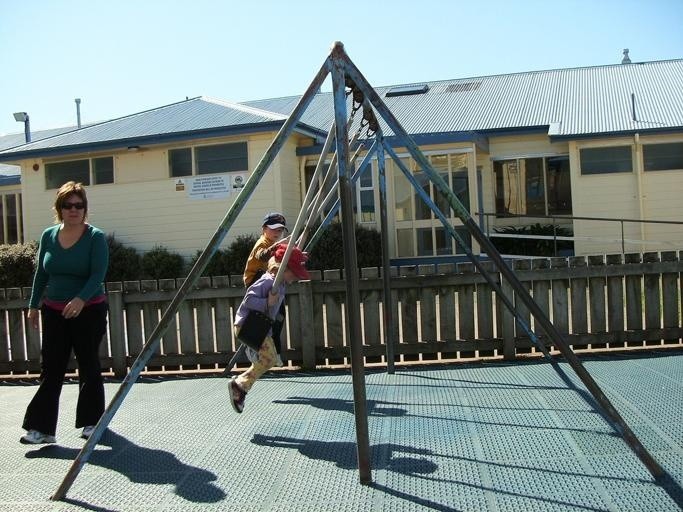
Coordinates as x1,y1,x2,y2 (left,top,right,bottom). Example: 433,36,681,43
275,353,284,367
82,425,95,439
227,378,247,414
19,428,56,444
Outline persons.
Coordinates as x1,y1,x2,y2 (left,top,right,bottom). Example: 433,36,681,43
17,179,111,445
241,213,290,367
225,243,314,415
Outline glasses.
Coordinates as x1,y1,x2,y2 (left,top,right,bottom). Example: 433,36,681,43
63,202,85,209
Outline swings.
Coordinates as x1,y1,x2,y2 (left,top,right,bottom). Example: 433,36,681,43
237,87,376,354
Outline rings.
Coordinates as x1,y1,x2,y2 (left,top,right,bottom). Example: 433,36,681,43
73,309,77,315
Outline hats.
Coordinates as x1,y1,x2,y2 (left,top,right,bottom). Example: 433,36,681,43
272,244,311,280
263,213,289,233
